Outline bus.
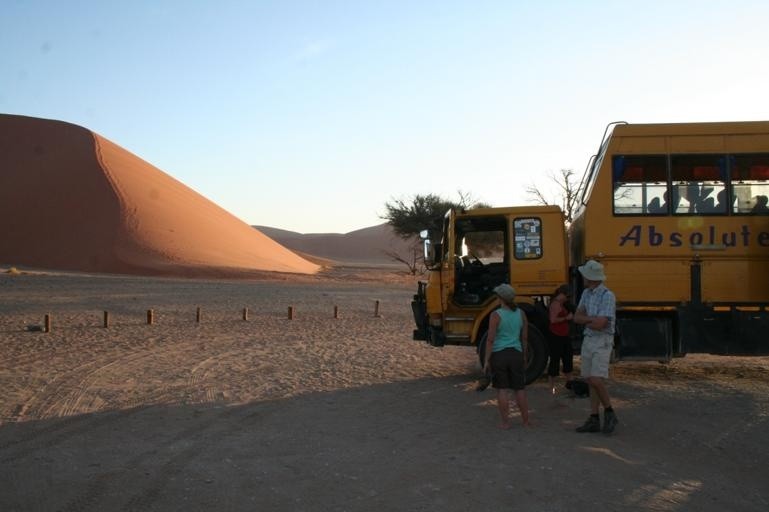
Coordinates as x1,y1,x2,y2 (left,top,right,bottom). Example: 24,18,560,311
585,257,590,258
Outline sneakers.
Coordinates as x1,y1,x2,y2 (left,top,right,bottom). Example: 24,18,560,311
576,416,601,432
603,411,619,433
476,376,491,392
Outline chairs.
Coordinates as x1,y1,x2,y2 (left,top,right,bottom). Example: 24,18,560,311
649,183,768,214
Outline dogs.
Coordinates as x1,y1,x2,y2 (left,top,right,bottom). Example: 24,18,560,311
565,380,589,399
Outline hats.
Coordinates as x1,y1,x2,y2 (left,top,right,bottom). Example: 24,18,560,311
494,283,516,301
578,260,607,282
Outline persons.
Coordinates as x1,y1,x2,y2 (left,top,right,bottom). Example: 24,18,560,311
548,285,574,394
482,282,529,428
751,196,768,213
572,260,618,434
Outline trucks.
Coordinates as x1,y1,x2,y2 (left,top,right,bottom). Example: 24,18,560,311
410,121,769,388
